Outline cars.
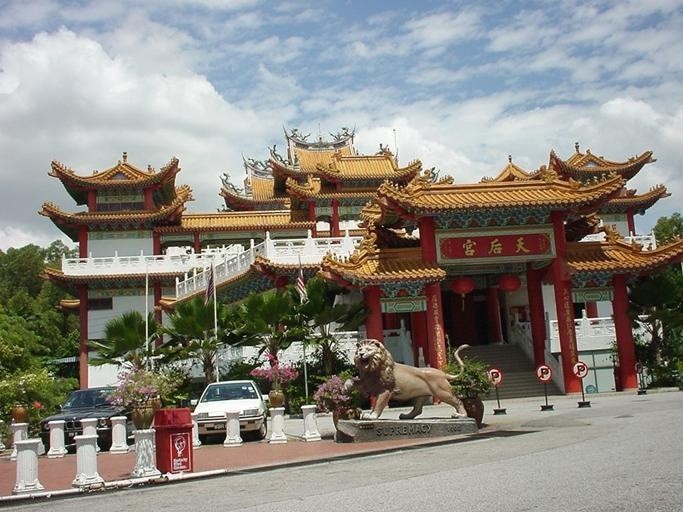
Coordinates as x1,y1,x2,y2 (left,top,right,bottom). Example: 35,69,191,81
193,377,269,445
38,388,137,455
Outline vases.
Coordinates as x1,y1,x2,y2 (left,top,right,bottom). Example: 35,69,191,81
332,411,348,429
10,403,28,423
267,389,284,407
130,406,153,429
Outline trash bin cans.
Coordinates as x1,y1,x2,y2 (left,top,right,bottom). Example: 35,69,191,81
152,407,195,474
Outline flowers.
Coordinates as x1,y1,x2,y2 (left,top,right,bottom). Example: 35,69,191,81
313,373,360,416
98,363,171,413
0,368,61,423
248,349,299,390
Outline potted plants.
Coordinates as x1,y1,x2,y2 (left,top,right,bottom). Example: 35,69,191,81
438,344,494,429
